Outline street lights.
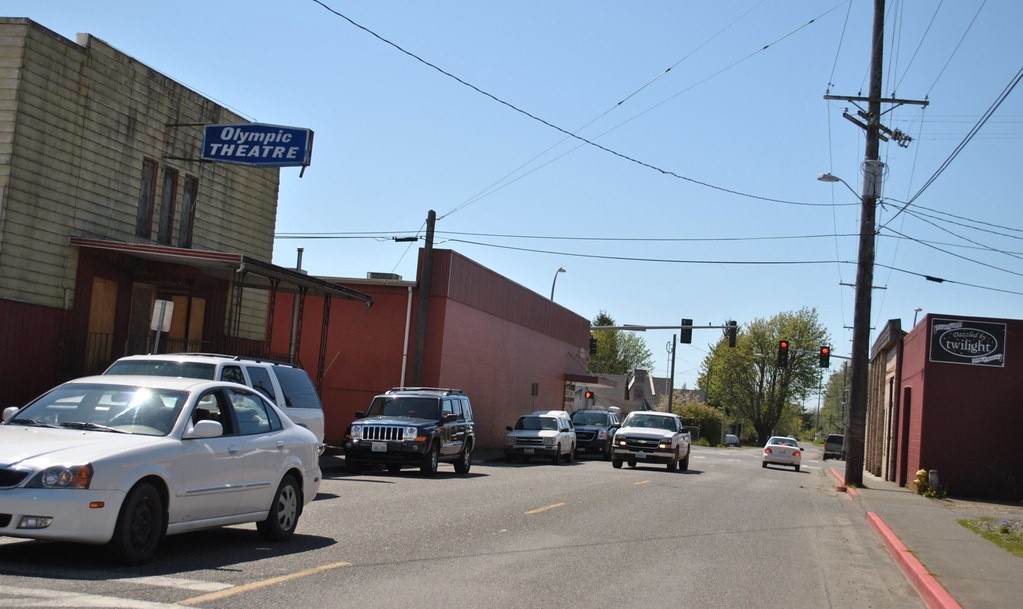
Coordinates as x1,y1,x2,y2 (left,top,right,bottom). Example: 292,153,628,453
816,174,885,486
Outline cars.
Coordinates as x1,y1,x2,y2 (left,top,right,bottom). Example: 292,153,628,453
0,375,323,560
762,436,804,472
726,434,739,446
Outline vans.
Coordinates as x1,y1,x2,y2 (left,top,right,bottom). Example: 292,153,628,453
823,433,844,460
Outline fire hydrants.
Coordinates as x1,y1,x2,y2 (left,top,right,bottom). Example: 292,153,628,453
913,469,929,495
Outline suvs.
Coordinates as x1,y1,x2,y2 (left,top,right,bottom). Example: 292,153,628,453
570,409,619,459
343,387,476,475
610,411,690,472
42,352,326,457
504,410,576,464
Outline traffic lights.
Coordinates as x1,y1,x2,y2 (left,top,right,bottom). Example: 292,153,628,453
777,340,788,368
585,392,593,399
819,346,829,368
